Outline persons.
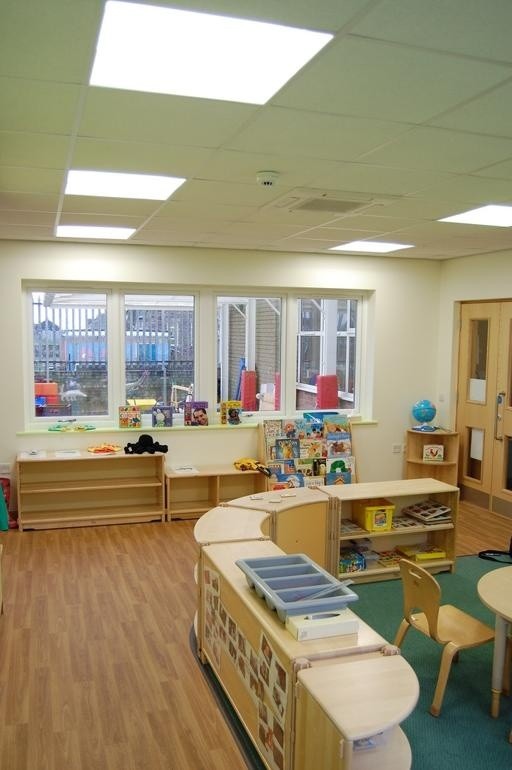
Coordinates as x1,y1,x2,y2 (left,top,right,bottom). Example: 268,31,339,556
190,407,208,426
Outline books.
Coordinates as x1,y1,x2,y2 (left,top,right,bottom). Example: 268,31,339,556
262,411,357,491
220,400,241,425
118,406,141,428
151,405,173,427
183,401,208,426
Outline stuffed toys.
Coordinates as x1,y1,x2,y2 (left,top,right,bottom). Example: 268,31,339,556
152,409,165,430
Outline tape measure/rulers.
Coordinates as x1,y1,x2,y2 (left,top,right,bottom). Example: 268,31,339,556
297,578,354,601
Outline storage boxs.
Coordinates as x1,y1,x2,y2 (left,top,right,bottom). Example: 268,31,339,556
352,498,395,531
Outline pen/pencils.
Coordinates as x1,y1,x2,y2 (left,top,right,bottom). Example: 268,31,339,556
304,614,340,620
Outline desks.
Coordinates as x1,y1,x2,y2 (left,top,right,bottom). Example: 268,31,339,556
478,566,512,718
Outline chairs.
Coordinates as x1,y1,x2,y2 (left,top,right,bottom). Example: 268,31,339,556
392,559,496,716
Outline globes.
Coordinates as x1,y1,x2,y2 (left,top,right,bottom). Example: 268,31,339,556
411,400,436,432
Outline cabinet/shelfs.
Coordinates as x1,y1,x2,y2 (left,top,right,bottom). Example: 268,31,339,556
15,447,165,532
164,469,267,521
192,477,460,770
257,422,361,491
403,428,460,486
